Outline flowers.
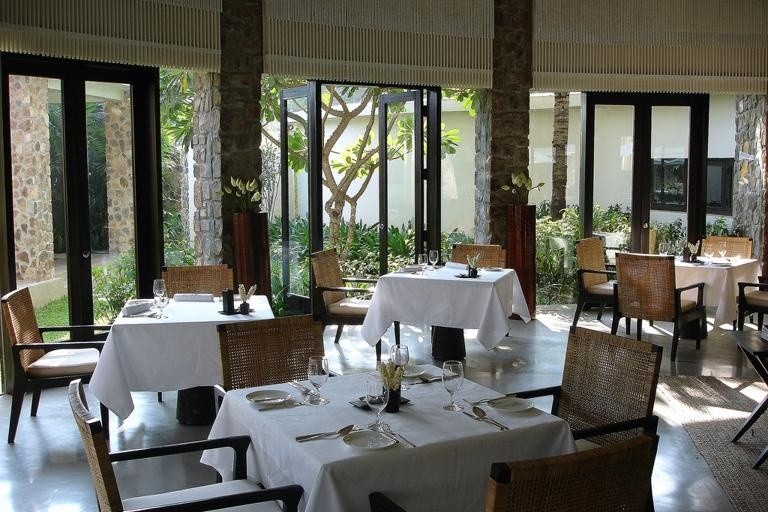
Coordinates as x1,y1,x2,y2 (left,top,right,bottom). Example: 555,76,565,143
215,175,262,212
501,170,545,193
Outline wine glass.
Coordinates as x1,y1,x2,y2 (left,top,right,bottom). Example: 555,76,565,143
429,250,438,272
153,279,166,296
154,288,168,319
418,254,428,278
659,239,728,266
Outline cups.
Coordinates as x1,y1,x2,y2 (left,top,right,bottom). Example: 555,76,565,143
308,344,465,431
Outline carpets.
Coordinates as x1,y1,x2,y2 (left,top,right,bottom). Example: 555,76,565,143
657,374,768,512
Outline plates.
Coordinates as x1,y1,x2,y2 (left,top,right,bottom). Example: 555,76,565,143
246,365,534,450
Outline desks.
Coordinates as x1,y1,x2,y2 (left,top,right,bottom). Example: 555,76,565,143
506,204,536,320
233,213,272,306
